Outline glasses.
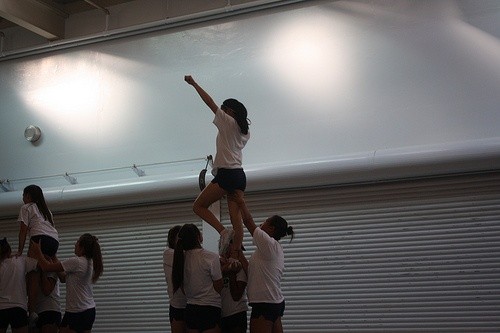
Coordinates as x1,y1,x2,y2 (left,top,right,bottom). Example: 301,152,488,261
221,105,227,110
264,217,274,230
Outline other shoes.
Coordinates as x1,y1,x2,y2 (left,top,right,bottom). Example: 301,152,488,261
217,227,234,258
220,258,239,278
29,312,39,330
58,271,66,283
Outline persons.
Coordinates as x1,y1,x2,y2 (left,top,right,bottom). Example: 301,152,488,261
0,185,61,333
232,190,292,333
29,233,102,333
185,76,250,256
163,224,247,333
